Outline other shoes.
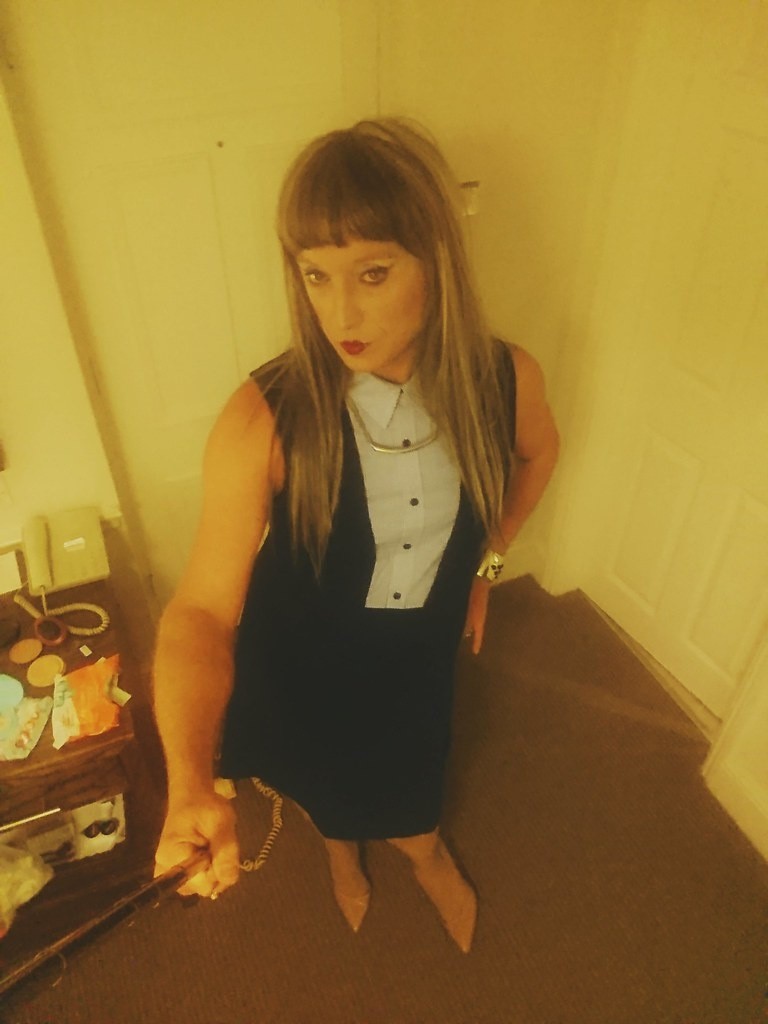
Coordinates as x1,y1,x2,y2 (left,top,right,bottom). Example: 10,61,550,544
414,837,476,954
327,844,371,933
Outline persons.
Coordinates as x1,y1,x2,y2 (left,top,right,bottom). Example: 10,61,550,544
151,115,559,957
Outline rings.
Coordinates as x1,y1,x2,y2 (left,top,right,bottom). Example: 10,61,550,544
464,634,471,637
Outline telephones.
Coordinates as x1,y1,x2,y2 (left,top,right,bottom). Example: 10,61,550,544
20,505,111,597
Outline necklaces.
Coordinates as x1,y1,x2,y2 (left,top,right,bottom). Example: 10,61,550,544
345,396,437,454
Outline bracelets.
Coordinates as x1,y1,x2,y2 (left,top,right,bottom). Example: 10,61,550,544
475,549,505,582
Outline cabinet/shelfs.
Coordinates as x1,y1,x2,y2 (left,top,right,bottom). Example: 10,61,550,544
0,578,195,926
